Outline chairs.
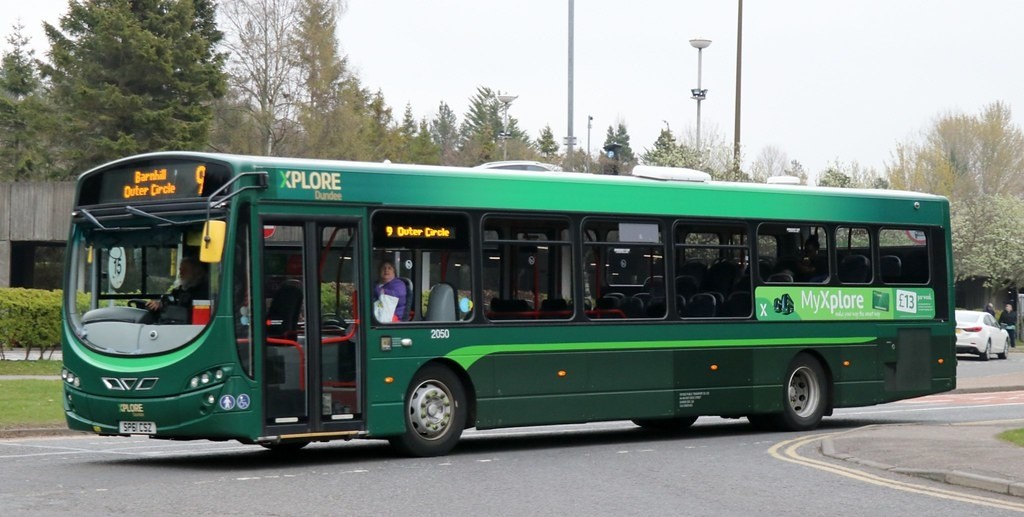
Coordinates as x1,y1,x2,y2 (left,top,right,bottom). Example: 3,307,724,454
490,255,900,315
268,279,302,346
397,277,414,321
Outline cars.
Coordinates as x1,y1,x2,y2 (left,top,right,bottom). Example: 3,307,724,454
956,310,1010,360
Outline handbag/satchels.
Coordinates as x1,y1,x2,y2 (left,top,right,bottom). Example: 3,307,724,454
373,289,400,325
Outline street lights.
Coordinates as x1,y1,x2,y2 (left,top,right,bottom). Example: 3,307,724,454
497,94,516,159
690,37,712,154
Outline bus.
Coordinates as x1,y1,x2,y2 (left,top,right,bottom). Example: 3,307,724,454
57,151,958,459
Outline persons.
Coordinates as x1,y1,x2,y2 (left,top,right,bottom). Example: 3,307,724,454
983,303,996,318
146,257,208,326
785,233,824,282
345,261,406,343
998,304,1017,349
235,287,288,339
